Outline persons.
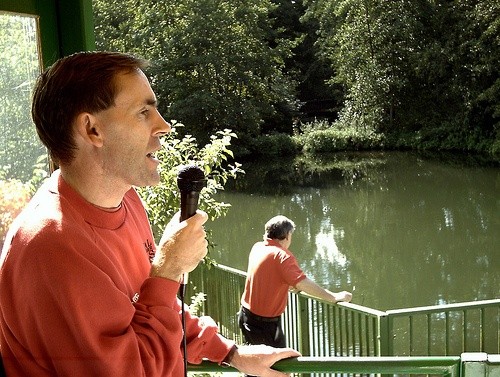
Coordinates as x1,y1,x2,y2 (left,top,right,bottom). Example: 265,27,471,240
0,51,302,377
236,215,352,348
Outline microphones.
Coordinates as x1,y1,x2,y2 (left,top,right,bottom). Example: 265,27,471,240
177,165,208,299
